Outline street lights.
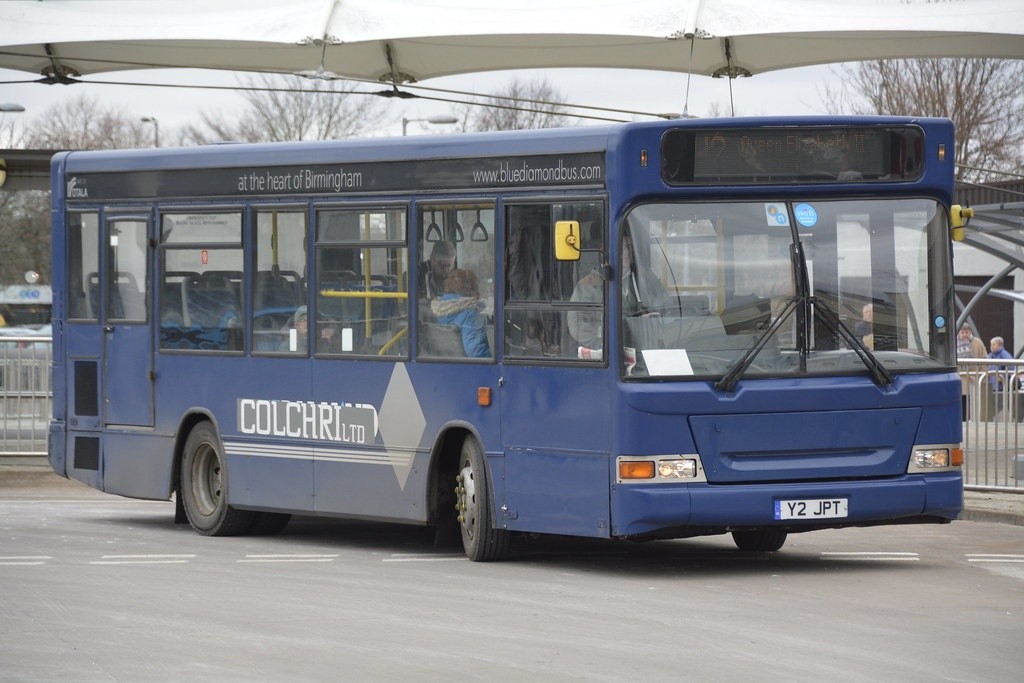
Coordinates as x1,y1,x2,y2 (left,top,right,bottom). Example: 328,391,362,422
140,116,159,148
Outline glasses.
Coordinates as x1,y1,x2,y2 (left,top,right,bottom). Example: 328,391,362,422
437,262,456,269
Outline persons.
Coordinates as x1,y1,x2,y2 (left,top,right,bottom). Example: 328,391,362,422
281,307,351,353
433,268,494,358
412,238,458,298
851,297,875,352
984,336,1017,412
567,234,678,352
953,321,988,421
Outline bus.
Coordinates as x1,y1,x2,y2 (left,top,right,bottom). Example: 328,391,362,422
43,111,978,563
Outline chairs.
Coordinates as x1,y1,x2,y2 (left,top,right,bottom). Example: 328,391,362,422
164,270,396,349
85,272,143,320
